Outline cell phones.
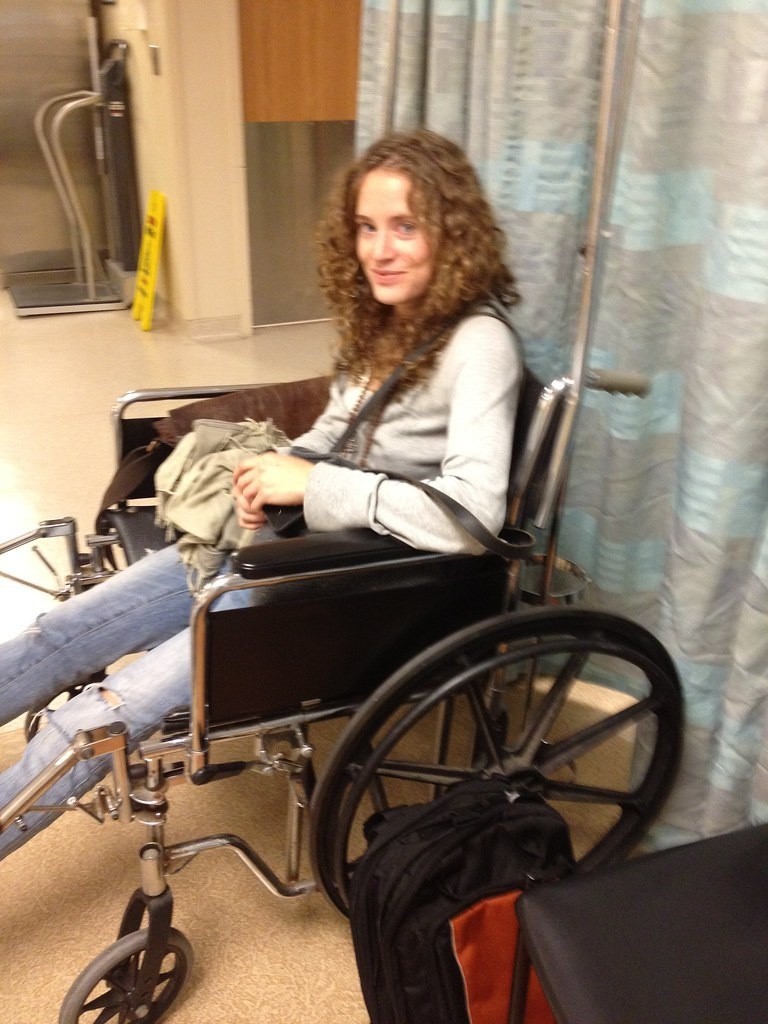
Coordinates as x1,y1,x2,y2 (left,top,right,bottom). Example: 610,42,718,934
260,503,303,534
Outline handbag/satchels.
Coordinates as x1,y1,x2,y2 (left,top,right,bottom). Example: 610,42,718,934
153,415,291,595
263,446,361,539
154,372,333,449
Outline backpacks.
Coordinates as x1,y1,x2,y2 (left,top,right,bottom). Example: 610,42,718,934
349,778,579,1024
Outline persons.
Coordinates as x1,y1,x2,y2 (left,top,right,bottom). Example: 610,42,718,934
0,128,522,859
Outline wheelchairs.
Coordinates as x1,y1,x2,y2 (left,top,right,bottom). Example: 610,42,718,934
0,364,687,1024
